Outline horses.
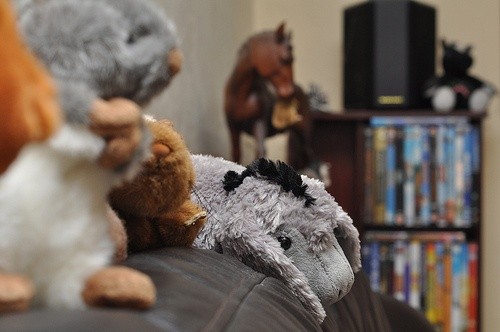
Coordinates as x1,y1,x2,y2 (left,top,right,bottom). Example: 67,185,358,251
222,20,328,184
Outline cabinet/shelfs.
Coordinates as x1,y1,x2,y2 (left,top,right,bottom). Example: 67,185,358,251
287,110,486,332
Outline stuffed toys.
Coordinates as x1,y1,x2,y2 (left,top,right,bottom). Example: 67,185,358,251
189,154,361,323
0,0,206,310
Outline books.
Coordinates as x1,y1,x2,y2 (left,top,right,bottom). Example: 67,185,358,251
365,126,478,227
361,242,479,332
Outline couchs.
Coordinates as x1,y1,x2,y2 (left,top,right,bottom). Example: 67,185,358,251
0,246,434,332
